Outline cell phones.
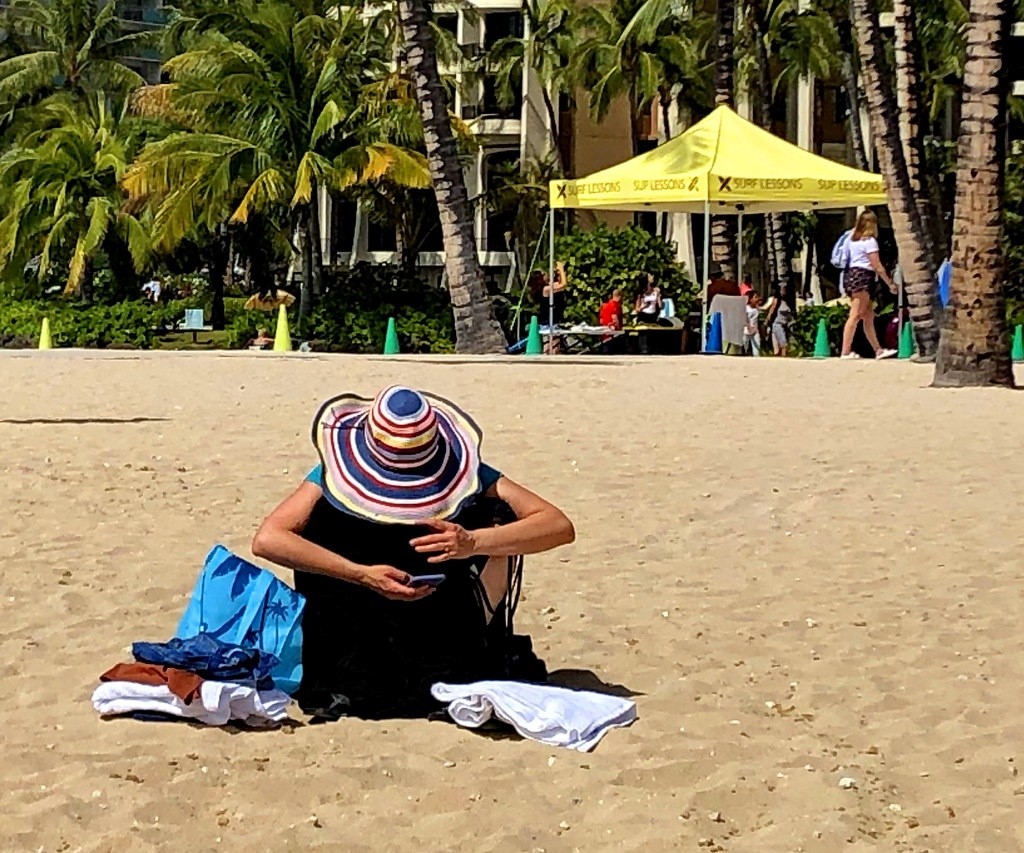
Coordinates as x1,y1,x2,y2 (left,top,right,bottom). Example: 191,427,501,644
407,574,446,589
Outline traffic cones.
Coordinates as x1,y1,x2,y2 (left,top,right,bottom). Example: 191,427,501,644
1010,324,1024,363
897,320,916,359
272,303,292,351
698,311,724,355
523,315,543,355
384,317,400,355
38,316,53,349
805,317,832,358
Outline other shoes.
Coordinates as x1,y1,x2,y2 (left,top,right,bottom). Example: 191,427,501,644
839,351,863,359
874,348,898,359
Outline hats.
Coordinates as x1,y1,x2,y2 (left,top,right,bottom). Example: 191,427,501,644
310,383,484,528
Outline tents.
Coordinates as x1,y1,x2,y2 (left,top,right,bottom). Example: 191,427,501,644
550,105,889,354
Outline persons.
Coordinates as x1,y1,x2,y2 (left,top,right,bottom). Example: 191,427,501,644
253,386,575,718
840,210,898,360
142,277,191,307
597,273,792,356
531,262,566,324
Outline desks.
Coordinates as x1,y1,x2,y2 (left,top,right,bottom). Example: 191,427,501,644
148,324,215,342
539,324,625,354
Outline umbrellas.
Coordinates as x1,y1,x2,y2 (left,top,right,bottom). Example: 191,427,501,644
243,289,296,311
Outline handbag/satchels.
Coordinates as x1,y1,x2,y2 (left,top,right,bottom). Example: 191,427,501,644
175,546,307,698
